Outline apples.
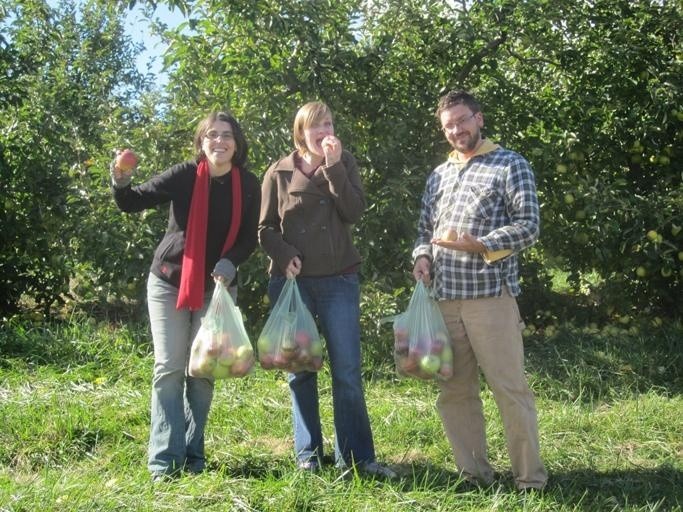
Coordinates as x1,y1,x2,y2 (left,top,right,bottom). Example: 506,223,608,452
257,332,323,369
189,332,254,377
522,108,683,339
117,149,136,170
442,229,457,241
395,325,453,380
321,135,336,149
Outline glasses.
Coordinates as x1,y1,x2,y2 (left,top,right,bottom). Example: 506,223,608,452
441,112,477,132
205,132,233,140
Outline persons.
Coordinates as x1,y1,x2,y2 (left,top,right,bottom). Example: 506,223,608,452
258,101,397,480
110,111,261,482
411,90,549,495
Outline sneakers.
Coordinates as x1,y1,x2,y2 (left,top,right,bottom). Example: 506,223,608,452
364,463,398,479
519,485,549,500
299,460,318,469
455,481,477,493
